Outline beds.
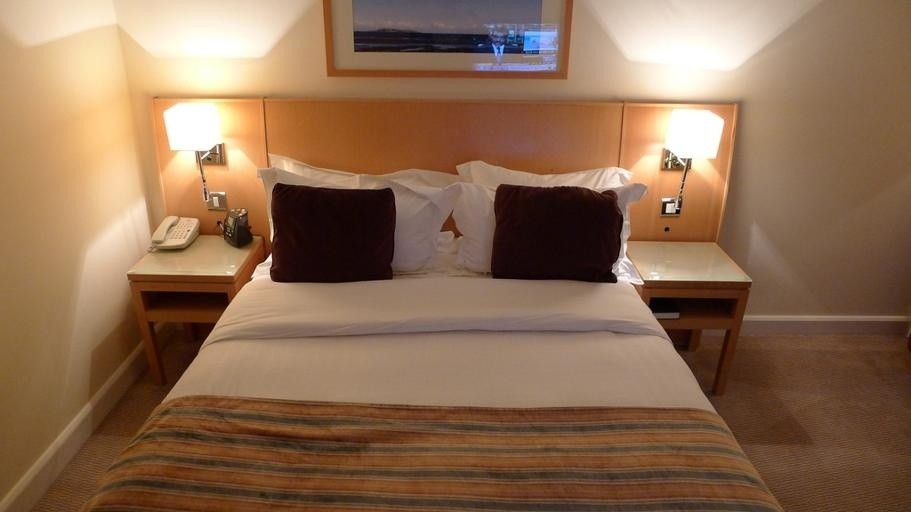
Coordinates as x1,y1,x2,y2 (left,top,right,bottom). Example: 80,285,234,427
85,95,784,512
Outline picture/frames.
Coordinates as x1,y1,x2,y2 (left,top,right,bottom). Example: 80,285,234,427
321,0,574,82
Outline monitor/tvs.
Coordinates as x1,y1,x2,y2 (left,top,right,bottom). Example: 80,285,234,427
465,19,561,71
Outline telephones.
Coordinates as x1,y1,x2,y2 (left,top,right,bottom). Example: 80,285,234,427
147,215,200,253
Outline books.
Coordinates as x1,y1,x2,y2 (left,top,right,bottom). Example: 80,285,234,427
651,298,681,321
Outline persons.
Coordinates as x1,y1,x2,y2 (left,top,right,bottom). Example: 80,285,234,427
477,24,523,64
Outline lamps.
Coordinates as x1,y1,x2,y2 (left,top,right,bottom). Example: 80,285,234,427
158,97,232,203
653,106,725,211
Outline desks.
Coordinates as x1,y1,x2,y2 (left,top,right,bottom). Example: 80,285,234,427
125,232,263,387
622,240,752,396
